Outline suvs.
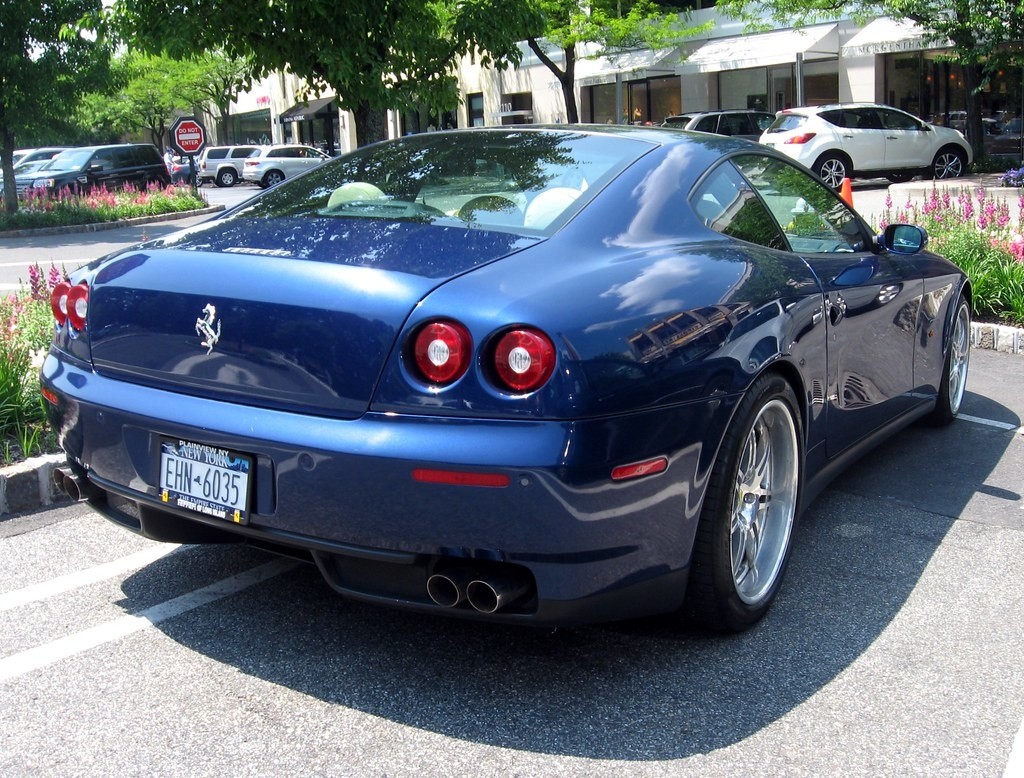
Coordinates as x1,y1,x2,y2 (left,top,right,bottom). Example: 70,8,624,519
0,143,172,207
654,107,777,143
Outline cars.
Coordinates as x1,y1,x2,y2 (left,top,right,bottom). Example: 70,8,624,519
37,122,975,632
757,102,974,193
170,145,337,189
940,111,1000,134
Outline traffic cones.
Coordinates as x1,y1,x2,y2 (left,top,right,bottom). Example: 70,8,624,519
838,178,853,209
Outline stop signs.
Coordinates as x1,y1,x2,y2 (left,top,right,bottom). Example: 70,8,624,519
175,120,205,153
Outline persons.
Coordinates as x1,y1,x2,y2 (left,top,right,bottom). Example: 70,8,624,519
164,150,172,175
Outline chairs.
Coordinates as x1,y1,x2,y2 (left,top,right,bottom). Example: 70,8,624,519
524,187,584,230
855,115,871,128
460,195,524,227
326,181,388,210
